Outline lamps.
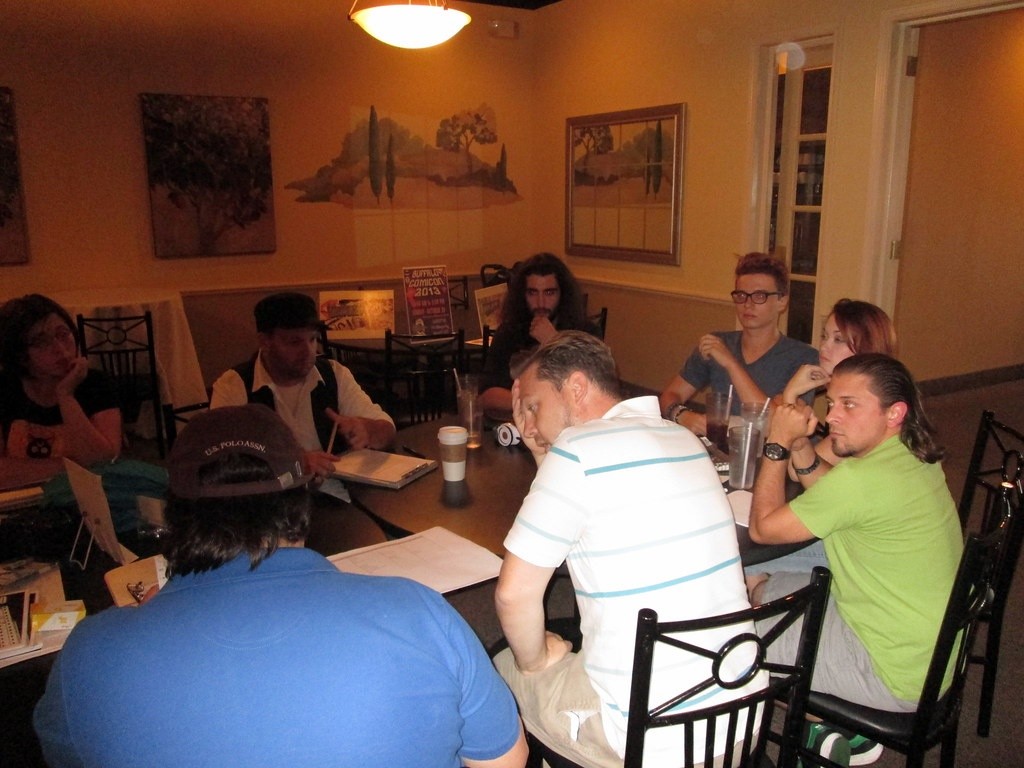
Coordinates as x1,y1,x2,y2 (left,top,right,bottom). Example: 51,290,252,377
347,0,472,50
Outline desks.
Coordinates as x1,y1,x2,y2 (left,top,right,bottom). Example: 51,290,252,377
0,490,390,702
317,330,483,397
348,415,802,657
50,289,209,439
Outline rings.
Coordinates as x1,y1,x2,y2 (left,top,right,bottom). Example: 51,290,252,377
788,404,795,409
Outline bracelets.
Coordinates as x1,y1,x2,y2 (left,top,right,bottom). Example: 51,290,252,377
792,452,820,475
666,402,694,424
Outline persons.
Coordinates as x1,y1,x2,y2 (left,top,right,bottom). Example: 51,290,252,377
774,298,897,482
491,331,770,768
745,354,975,768
479,253,602,415
660,251,822,437
210,293,396,486
0,293,126,493
33,405,530,768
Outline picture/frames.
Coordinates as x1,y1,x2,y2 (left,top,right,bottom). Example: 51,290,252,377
564,102,689,269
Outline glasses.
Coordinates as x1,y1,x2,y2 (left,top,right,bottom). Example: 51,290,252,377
731,290,785,304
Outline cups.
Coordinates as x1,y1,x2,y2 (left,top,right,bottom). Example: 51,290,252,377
740,401,770,457
705,393,733,444
438,426,469,482
729,426,760,490
454,375,484,448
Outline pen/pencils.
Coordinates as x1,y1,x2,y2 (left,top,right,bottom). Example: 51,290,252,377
323,421,341,459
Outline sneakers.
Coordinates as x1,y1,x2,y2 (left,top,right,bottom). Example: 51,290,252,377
840,727,884,767
800,722,852,768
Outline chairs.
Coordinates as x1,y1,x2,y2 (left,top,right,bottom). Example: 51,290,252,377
477,323,496,395
76,309,166,461
958,409,1024,739
769,481,1016,768
377,327,465,431
418,273,480,369
526,566,832,768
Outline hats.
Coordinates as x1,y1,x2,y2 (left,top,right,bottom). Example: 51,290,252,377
255,293,325,329
168,406,314,496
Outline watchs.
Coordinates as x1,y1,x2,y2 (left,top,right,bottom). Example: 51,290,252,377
763,442,791,460
